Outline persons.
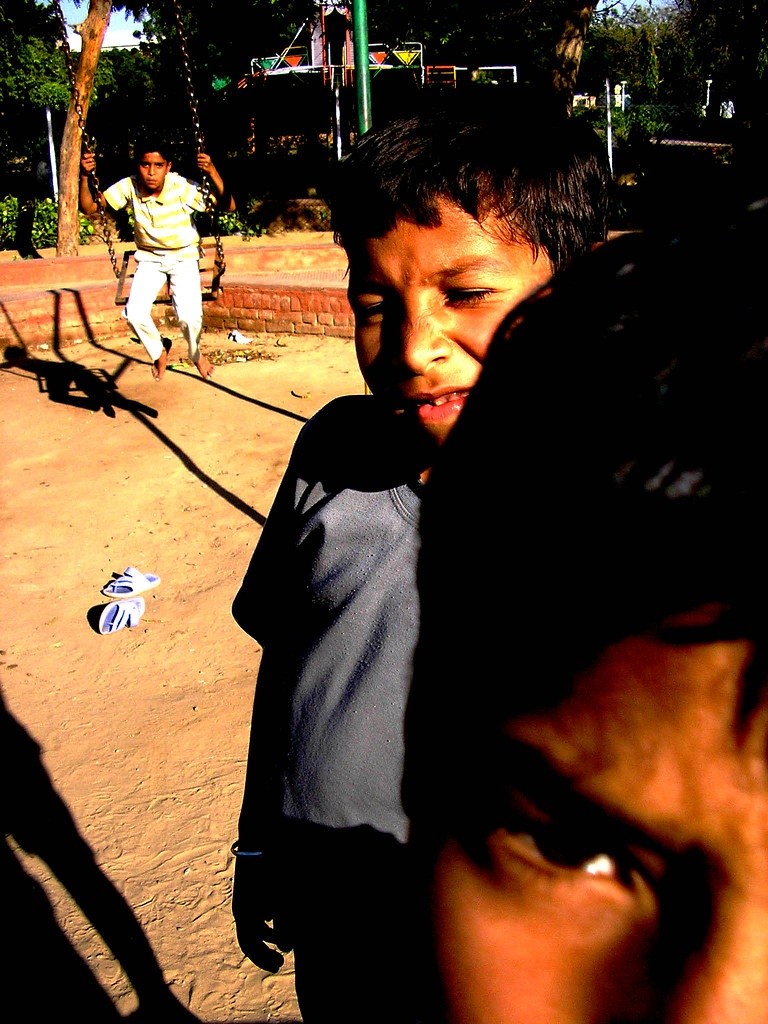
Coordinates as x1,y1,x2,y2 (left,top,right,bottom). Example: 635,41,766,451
79,147,236,383
233,84,768,1024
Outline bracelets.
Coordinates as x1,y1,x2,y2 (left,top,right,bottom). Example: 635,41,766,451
231,838,267,857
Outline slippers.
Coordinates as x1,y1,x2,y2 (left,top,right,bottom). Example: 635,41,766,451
98,596,145,635
101,566,161,598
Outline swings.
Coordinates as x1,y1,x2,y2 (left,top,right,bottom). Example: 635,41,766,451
50,1,227,309
